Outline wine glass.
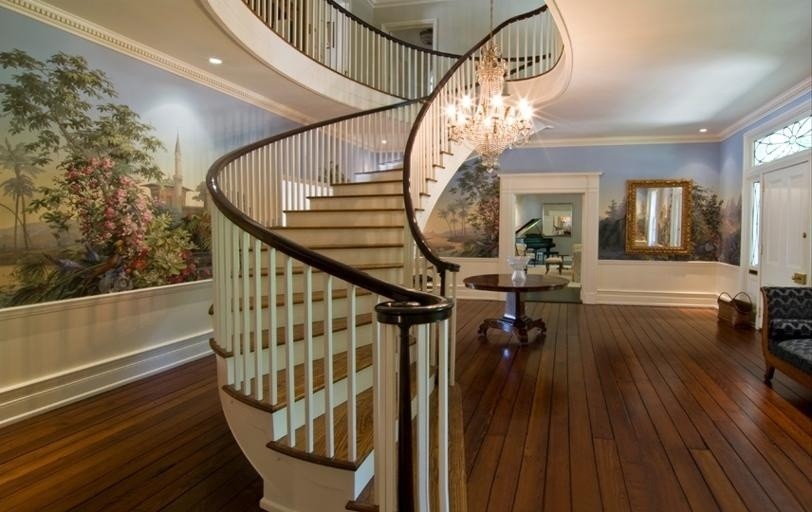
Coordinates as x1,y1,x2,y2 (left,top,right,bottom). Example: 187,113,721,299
506,255,532,281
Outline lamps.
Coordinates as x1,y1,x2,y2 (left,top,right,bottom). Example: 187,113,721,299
445,0,534,172
420,29,432,45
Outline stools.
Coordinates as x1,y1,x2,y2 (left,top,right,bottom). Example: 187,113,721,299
544,258,562,274
543,252,559,264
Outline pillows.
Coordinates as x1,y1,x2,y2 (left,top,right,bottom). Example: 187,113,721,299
770,319,812,340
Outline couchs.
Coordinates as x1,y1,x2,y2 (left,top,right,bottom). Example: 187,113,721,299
760,286,812,394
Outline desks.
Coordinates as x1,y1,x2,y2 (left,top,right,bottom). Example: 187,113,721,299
572,243,582,282
556,255,569,270
464,274,569,348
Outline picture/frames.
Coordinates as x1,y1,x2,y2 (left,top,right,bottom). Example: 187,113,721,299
625,179,692,255
542,202,573,237
